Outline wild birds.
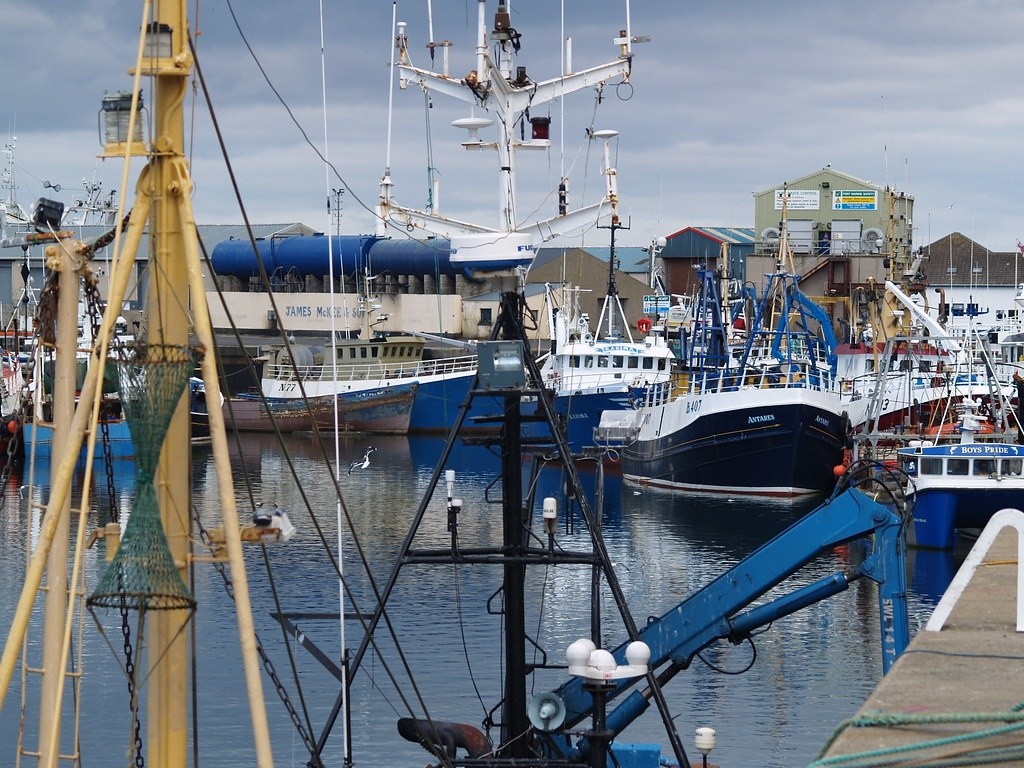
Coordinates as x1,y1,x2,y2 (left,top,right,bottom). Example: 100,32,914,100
347,445,378,477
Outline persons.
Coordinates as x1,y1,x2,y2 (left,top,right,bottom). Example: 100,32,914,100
822,233,829,254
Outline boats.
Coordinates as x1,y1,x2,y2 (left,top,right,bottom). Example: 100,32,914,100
0,0,1024,768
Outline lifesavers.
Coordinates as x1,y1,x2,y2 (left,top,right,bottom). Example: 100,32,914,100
637,318,651,333
